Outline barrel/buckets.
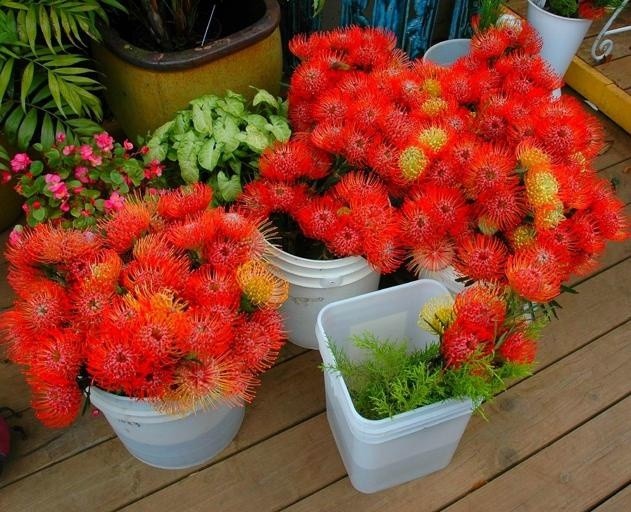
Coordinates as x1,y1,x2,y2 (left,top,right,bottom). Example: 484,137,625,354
73,325,253,474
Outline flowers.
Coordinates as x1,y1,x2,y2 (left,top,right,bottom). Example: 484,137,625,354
0,182,293,429
317,278,538,414
545,0,628,18
236,17,629,302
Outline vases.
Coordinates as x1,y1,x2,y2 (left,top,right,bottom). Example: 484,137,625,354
527,0,592,101
80,379,244,470
91,2,283,138
422,35,472,69
